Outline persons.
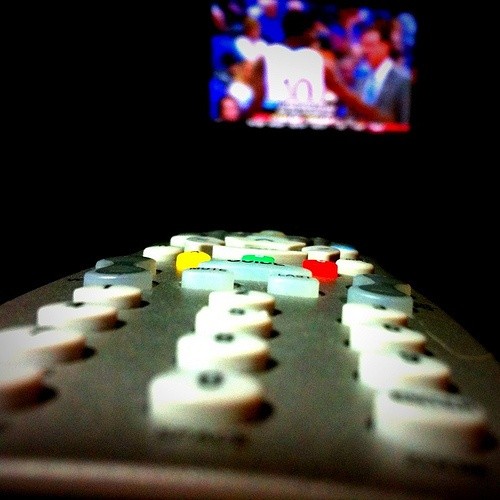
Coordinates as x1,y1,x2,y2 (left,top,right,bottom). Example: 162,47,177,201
208,2,418,130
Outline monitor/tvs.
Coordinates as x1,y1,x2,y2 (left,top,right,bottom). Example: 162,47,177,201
201,0,420,136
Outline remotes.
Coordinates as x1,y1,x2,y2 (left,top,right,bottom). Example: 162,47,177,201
0,229,500,500
